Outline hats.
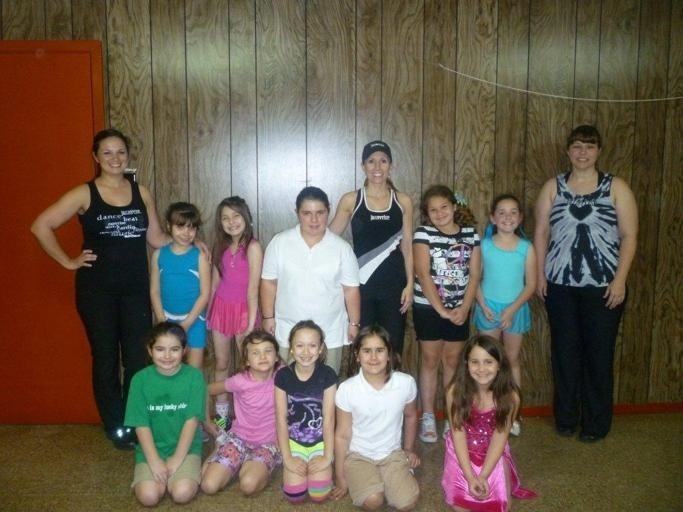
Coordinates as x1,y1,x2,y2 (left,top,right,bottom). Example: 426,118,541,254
362,141,391,165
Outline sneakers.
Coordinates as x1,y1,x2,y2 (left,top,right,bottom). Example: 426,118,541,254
419,413,437,443
199,415,228,441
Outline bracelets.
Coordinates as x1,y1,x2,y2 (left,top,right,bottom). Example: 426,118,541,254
350,321,361,328
261,315,274,319
156,316,168,321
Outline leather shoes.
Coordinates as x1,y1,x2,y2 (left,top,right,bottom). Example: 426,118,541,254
559,424,574,436
108,426,137,449
578,430,597,441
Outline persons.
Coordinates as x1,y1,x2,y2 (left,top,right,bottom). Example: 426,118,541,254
331,325,422,511
259,186,361,378
199,329,287,496
326,140,415,376
149,201,214,443
274,320,339,503
31,128,212,451
124,321,208,507
474,192,538,436
533,124,640,444
204,195,264,448
441,334,538,512
412,184,481,445
